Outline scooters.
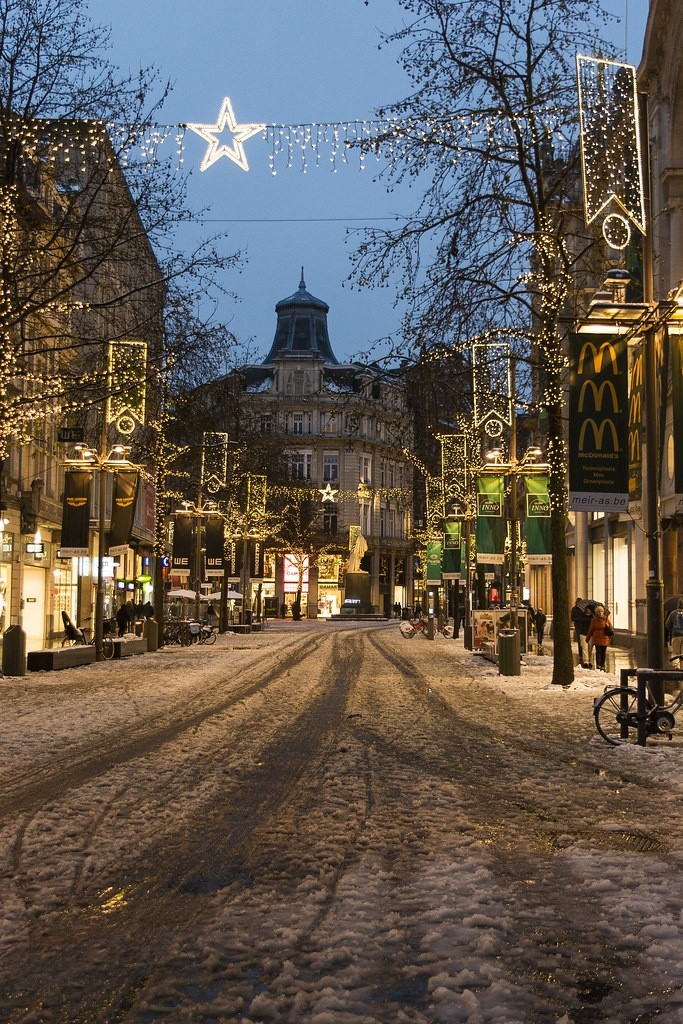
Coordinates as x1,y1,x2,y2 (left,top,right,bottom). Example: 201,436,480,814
400,611,453,639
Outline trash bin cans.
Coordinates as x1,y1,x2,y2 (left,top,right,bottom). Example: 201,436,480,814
497,627,521,676
2,624,26,676
246,609,253,632
144,618,158,651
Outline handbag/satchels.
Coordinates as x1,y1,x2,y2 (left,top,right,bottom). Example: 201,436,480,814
604,617,614,636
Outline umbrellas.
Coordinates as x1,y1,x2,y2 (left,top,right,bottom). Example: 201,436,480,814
663,594,683,614
577,600,610,616
167,589,249,601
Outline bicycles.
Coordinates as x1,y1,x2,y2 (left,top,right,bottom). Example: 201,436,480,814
165,616,217,646
591,650,683,747
60,614,116,660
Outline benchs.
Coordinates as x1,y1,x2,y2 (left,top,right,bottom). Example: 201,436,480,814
102,637,147,659
28,645,94,671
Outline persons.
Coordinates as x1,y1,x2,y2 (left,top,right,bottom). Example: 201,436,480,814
393,601,401,619
458,603,465,628
532,608,546,646
571,598,613,672
116,599,154,637
280,601,288,619
665,597,683,670
414,600,422,622
207,601,217,625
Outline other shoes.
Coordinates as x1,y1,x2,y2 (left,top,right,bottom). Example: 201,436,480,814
589,663,592,670
582,663,588,668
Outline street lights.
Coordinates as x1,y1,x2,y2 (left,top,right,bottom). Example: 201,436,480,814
74,443,132,663
483,443,544,631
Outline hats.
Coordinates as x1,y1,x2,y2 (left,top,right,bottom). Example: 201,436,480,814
595,606,605,614
